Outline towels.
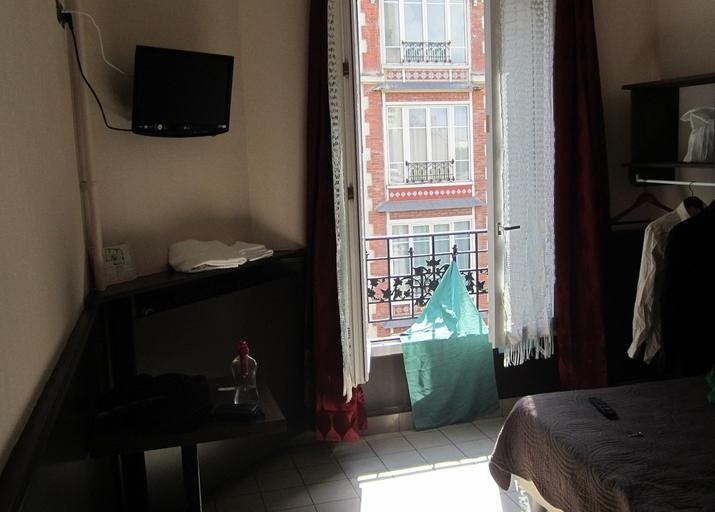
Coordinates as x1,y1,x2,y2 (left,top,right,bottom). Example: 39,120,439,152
168,241,274,275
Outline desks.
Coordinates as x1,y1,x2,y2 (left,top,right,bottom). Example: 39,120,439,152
39,372,289,512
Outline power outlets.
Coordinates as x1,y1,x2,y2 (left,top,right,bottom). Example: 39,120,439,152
57,0,67,29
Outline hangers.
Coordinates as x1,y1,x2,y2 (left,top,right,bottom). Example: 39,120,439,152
609,179,708,225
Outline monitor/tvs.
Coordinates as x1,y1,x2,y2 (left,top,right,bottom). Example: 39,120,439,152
130,42,236,139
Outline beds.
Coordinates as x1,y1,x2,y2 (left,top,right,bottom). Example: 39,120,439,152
489,377,715,512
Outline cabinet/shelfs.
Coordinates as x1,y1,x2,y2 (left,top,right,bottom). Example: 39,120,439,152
620,72,714,186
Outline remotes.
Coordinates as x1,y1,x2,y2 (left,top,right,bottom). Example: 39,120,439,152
588,395,621,421
